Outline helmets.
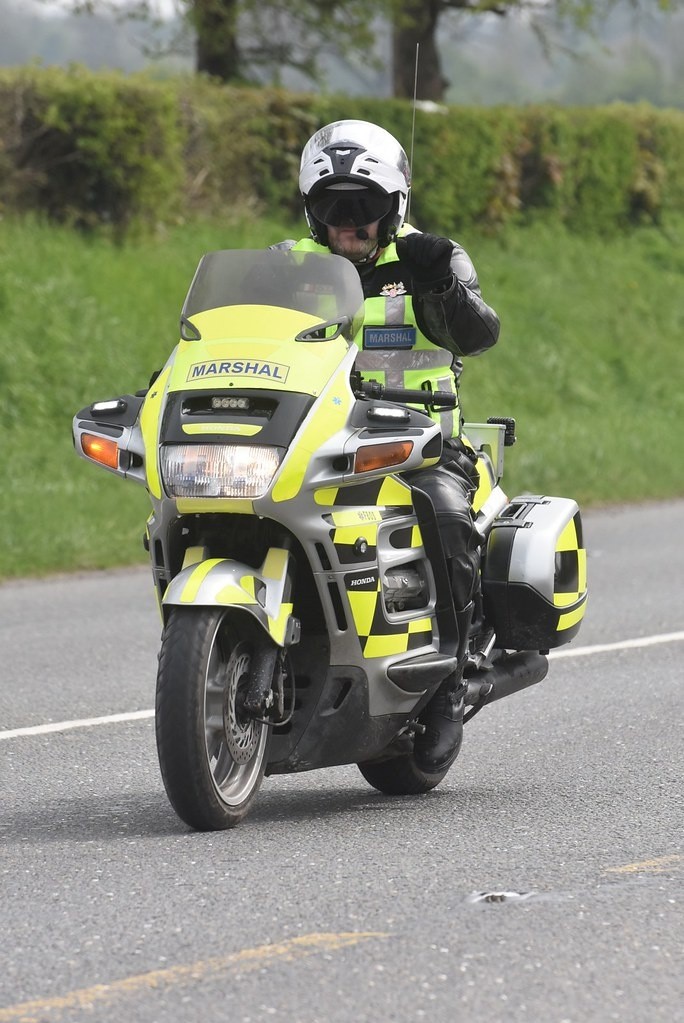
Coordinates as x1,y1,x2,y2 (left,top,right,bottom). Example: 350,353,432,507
298,119,411,247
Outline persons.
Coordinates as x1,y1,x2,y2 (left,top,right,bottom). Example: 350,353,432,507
148,119,501,767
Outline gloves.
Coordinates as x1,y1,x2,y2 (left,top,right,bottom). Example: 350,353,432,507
396,233,456,293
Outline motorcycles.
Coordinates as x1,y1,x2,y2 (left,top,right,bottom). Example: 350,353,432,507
69,248,594,829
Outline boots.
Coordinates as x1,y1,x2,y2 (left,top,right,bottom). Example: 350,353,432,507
414,602,476,775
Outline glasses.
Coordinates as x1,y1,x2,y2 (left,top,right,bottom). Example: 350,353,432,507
309,187,397,230
